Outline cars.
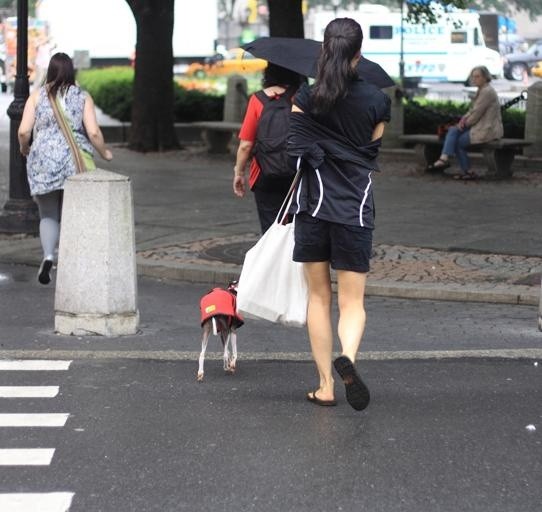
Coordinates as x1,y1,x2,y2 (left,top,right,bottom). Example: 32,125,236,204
503,37,542,82
186,47,269,80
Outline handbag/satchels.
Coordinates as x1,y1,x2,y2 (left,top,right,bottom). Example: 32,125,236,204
46,81,96,173
233,218,311,330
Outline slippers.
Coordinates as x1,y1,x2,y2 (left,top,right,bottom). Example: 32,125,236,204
307,388,339,407
332,355,378,413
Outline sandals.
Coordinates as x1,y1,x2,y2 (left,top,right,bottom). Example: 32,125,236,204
427,160,449,172
454,171,479,180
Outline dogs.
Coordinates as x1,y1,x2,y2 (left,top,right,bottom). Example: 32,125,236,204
197,279,245,382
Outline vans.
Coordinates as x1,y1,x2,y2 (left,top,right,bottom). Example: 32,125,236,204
302,6,502,87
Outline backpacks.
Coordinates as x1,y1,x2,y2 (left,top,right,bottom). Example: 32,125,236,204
253,85,302,177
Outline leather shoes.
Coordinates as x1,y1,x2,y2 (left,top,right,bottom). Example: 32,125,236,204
37,254,58,286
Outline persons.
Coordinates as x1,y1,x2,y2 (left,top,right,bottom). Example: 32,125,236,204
284,16,393,412
0,0,58,97
232,58,306,237
18,53,115,284
424,66,505,175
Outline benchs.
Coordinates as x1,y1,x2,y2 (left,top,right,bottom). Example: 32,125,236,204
397,132,533,179
175,122,241,154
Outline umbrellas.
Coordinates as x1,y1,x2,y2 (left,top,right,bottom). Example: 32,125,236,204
239,36,397,90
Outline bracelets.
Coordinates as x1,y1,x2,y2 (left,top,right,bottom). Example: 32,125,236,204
233,166,245,177
